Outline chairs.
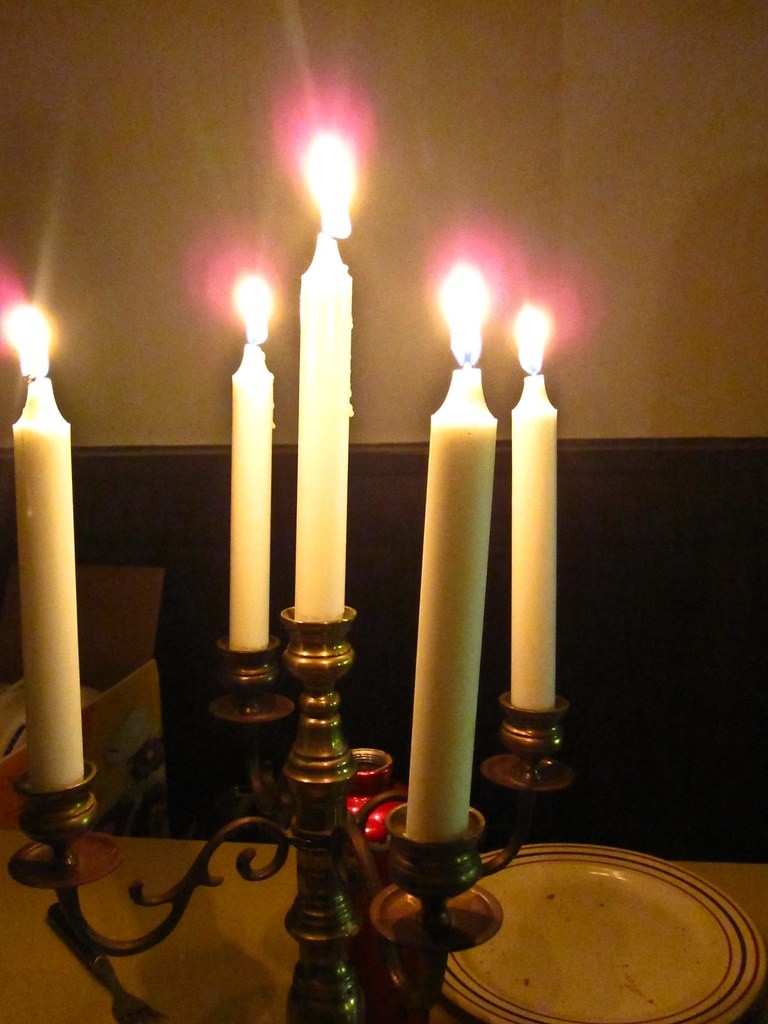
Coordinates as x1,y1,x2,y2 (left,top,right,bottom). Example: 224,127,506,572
1,657,170,839
1,562,166,692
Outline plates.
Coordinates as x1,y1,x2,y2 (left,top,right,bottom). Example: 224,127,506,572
441,843,768,1024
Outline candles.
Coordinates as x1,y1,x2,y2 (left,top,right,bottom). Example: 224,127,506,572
507,302,563,707
229,270,277,651
9,305,84,788
289,128,359,624
400,263,501,840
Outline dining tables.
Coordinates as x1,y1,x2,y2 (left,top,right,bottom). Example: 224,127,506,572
0,829,768,1024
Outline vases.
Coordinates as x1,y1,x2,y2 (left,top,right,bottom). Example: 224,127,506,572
335,748,405,1024
